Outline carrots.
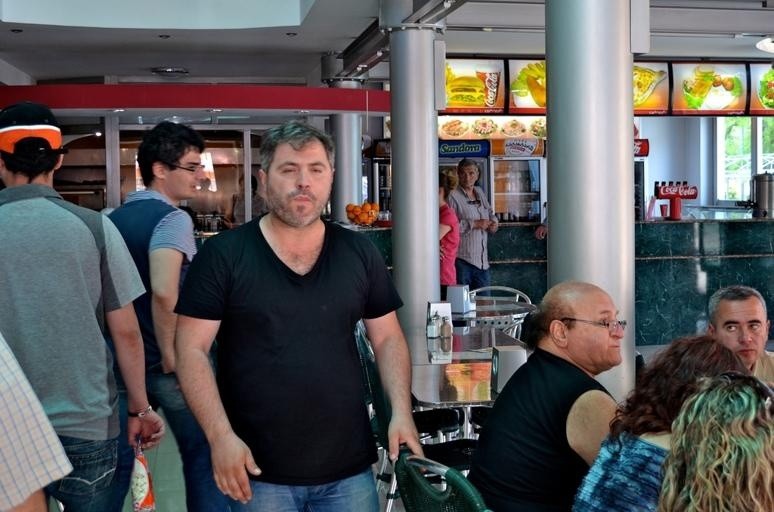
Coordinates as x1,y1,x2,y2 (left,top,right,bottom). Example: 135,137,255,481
526,75,547,107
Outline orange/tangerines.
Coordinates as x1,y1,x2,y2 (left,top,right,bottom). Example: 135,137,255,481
346,203,380,225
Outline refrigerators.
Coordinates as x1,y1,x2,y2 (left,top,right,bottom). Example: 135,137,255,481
438,140,489,205
371,139,392,211
634,126,649,221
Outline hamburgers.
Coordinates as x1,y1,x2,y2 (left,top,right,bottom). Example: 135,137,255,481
447,76,486,107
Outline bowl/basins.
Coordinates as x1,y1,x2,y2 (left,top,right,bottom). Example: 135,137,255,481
347,211,379,227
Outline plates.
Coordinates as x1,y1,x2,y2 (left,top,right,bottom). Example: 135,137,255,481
757,68,774,110
440,123,546,139
682,68,737,110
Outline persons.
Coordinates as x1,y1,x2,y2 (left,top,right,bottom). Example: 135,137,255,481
0,100,167,512
0,332,75,512
223,174,264,229
468,282,627,512
439,173,460,301
105,120,234,512
171,121,426,512
535,218,548,239
573,285,773,511
447,159,498,296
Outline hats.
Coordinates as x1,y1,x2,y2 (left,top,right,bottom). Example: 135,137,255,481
1,127,61,155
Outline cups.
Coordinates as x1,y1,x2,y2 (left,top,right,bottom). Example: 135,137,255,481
474,65,502,107
659,204,668,217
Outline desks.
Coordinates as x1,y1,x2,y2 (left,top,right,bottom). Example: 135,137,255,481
430,301,536,439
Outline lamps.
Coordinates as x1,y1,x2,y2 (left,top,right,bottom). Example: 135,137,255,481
755,35,774,53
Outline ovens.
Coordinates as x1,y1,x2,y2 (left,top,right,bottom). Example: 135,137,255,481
54,185,109,213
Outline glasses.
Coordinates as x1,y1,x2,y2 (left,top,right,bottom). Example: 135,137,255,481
562,317,627,331
157,161,206,173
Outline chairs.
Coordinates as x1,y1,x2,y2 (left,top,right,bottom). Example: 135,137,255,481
470,286,531,302
356,326,492,512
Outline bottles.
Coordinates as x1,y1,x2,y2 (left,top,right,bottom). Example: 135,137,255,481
493,160,540,222
427,338,441,360
427,315,440,338
440,316,451,339
131,435,155,511
440,338,452,356
469,293,476,311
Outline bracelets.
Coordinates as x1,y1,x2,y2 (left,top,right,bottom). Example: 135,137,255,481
230,223,232,228
127,406,152,418
